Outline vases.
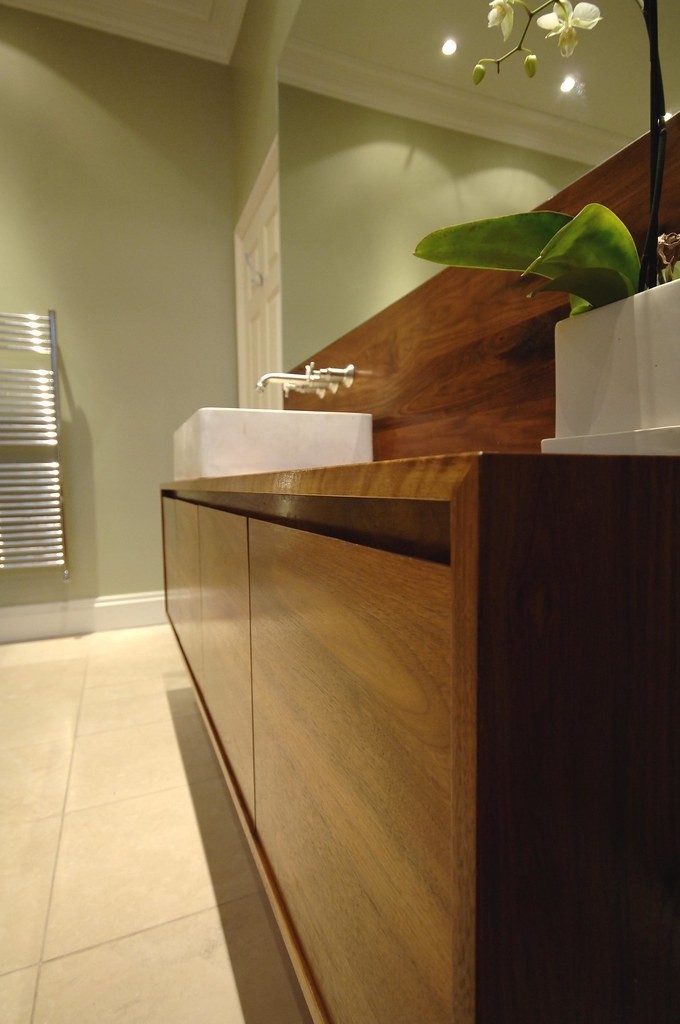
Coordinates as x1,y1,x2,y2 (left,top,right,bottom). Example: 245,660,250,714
554,281,678,435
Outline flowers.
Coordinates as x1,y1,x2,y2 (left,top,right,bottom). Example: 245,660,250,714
412,0,679,314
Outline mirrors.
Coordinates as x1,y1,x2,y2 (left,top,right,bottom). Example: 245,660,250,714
278,0,679,454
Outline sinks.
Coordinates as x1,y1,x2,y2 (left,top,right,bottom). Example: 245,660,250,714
173,407,373,482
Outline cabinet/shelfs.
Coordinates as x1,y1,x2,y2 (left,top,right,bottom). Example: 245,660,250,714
160,447,680,1023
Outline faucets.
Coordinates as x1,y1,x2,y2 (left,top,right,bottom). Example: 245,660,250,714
256,373,339,394
